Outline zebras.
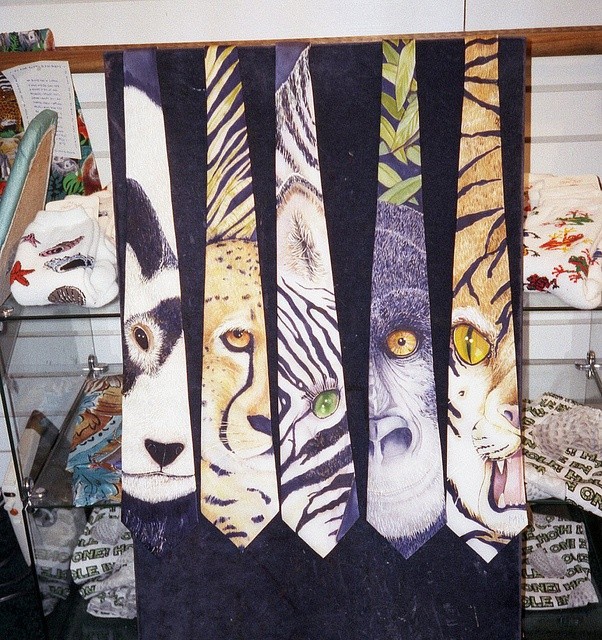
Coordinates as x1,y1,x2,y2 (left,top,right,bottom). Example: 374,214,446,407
280,45,362,557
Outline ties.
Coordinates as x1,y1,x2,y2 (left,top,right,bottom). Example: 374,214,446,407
366,40,445,559
121,48,198,557
274,42,360,559
198,47,279,552
457,36,529,562
